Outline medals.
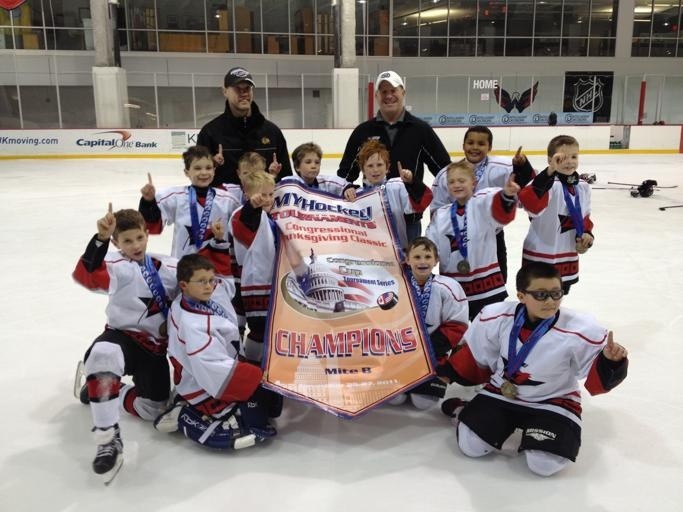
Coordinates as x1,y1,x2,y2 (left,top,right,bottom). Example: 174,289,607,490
558,176,590,254
500,307,555,401
135,256,171,339
450,201,470,275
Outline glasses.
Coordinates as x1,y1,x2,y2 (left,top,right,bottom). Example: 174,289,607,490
192,278,217,286
525,289,565,301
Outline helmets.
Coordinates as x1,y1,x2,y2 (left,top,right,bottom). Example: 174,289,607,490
639,180,657,197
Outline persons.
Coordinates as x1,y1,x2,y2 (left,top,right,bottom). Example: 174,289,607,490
440,261,627,477
356,140,432,265
337,71,452,242
213,144,267,202
425,163,520,320
137,146,241,262
73,204,177,484
269,143,356,201
405,235,470,409
195,66,293,191
153,219,284,452
228,168,282,364
518,135,594,296
429,124,536,222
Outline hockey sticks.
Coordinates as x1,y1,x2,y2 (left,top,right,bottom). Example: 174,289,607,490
591,186,661,192
607,181,678,189
658,205,682,211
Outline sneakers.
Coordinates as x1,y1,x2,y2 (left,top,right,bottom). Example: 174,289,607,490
80,384,89,404
93,425,123,474
441,398,470,417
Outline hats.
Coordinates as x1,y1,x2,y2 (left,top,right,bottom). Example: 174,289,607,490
224,67,255,88
375,71,405,91
